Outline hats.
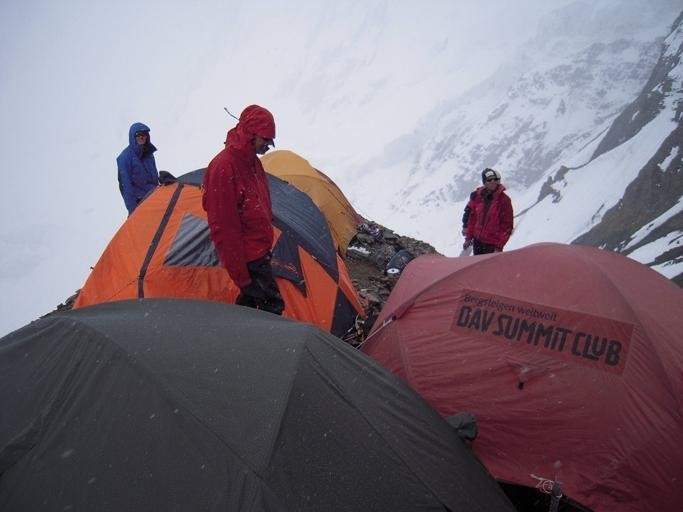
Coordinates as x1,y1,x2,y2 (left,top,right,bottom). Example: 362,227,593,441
482,168,497,184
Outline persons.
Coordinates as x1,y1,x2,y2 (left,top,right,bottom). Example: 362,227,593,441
117,123,159,214
202,105,285,315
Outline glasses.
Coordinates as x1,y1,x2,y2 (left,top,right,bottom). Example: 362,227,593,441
135,132,146,137
485,178,500,183
